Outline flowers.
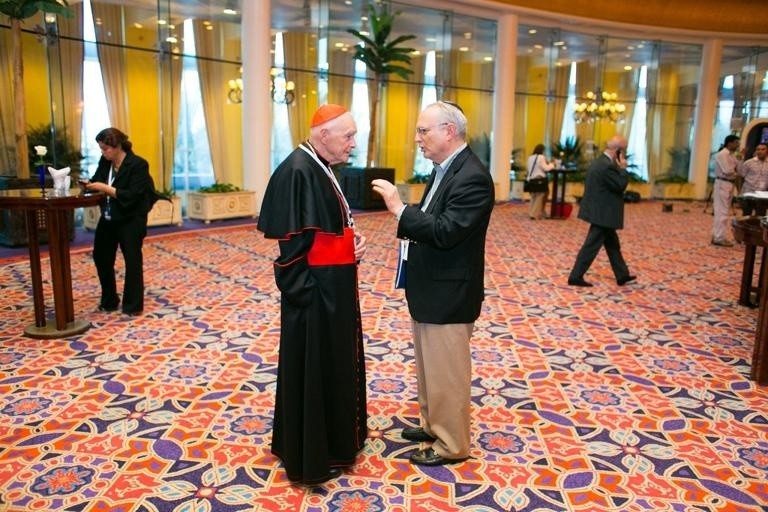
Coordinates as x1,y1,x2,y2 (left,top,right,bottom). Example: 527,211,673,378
31,146,52,167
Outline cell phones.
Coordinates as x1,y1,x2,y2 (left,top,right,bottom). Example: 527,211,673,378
616,148,621,162
78,181,87,186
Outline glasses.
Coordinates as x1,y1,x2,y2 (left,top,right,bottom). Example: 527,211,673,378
415,122,447,135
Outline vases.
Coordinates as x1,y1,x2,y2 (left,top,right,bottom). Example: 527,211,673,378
38,165,46,193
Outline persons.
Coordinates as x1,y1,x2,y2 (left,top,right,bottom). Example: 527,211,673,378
568,136,637,287
255,104,368,488
525,144,558,221
710,135,768,247
79,127,158,320
370,102,494,466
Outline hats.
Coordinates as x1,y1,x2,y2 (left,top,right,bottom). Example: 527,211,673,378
310,103,347,128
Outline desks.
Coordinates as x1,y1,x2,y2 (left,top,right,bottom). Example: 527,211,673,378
0,188,104,339
724,194,767,309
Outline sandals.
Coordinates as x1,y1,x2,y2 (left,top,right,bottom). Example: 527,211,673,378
121,311,137,319
94,305,107,312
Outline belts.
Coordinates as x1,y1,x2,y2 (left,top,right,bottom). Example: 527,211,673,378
716,176,734,183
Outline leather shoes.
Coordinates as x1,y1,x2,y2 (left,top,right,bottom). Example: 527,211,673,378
410,448,468,467
328,467,341,478
401,427,435,443
616,276,635,286
567,280,591,286
712,239,733,246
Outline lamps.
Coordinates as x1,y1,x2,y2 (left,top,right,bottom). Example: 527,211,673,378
227,77,243,104
269,68,296,104
569,35,628,125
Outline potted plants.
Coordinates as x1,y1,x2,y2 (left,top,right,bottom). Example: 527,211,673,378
85,191,183,234
334,1,416,210
510,136,695,222
395,176,429,207
0,1,81,249
189,180,254,225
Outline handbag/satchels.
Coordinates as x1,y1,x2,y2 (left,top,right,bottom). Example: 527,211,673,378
528,177,547,193
